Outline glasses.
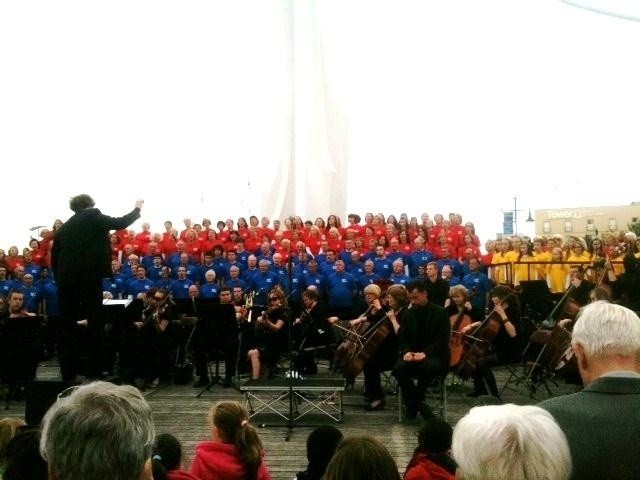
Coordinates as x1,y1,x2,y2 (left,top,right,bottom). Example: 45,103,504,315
153,296,161,300
269,297,278,301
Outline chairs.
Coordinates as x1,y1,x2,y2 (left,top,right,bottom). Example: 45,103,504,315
498,328,559,400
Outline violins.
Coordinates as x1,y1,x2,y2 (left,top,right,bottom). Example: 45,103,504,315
153,303,169,321
263,304,280,321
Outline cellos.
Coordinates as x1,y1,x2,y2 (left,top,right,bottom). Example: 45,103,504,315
343,294,412,376
448,286,479,370
333,292,387,369
550,335,577,374
459,284,523,371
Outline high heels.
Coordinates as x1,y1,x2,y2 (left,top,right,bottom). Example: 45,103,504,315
368,400,384,410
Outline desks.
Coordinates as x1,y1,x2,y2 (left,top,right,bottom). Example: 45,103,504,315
238,378,349,426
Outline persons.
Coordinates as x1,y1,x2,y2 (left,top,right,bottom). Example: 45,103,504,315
190,399,271,480
50,194,146,380
1,213,639,419
451,404,573,480
1,396,52,480
152,433,202,480
38,380,153,479
401,418,460,480
322,435,400,480
292,425,342,479
531,300,640,480
1,415,29,480
